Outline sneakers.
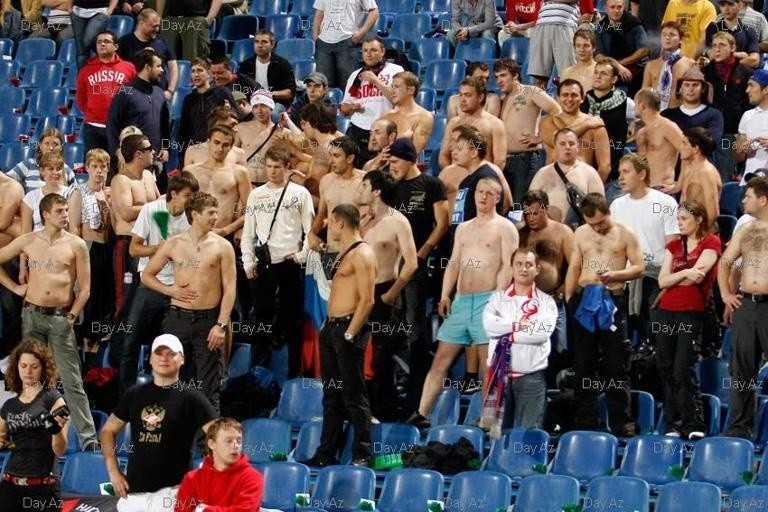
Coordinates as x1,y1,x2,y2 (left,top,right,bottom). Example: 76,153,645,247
610,422,636,436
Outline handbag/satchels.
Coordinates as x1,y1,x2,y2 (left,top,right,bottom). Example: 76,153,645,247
255,243,271,263
566,183,587,226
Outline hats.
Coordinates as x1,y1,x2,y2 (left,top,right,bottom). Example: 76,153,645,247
745,168,768,182
675,69,714,104
152,333,185,354
388,137,416,163
749,69,768,86
250,89,275,110
304,72,328,85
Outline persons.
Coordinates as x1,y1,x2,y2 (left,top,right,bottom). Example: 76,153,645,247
0,1,768,512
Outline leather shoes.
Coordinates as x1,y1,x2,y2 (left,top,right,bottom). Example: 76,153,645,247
298,455,337,466
689,431,705,440
666,431,680,438
352,458,368,466
718,428,752,439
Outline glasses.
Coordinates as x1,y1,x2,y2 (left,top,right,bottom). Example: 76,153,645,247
95,40,114,44
141,145,153,151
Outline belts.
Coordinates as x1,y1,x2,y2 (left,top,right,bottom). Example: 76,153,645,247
2,471,60,486
576,286,625,297
325,316,351,324
738,290,768,301
23,300,71,316
